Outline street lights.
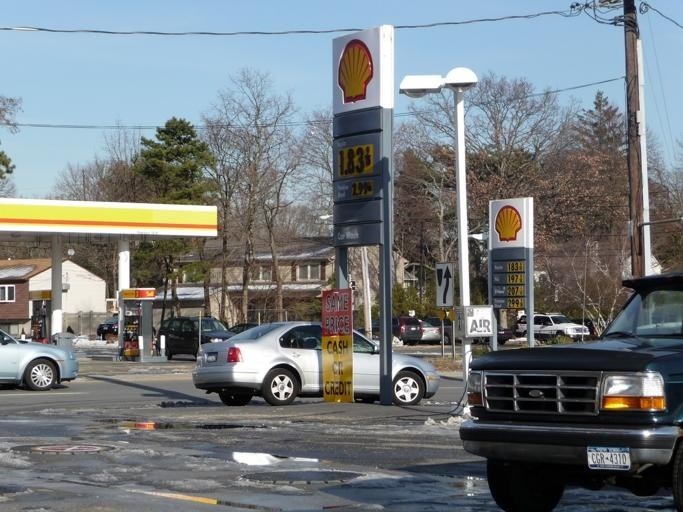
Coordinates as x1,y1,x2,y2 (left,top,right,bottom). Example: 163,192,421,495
399,67,478,416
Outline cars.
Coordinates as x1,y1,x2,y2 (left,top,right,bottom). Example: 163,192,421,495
473,324,513,344
571,318,594,335
191,320,441,407
356,316,440,344
0,329,80,391
228,323,260,333
424,315,454,345
97,316,118,340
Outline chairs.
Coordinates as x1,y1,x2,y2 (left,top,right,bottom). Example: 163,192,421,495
282,333,318,349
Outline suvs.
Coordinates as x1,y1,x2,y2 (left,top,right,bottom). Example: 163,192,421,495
456,272,683,512
515,312,590,342
156,315,238,360
